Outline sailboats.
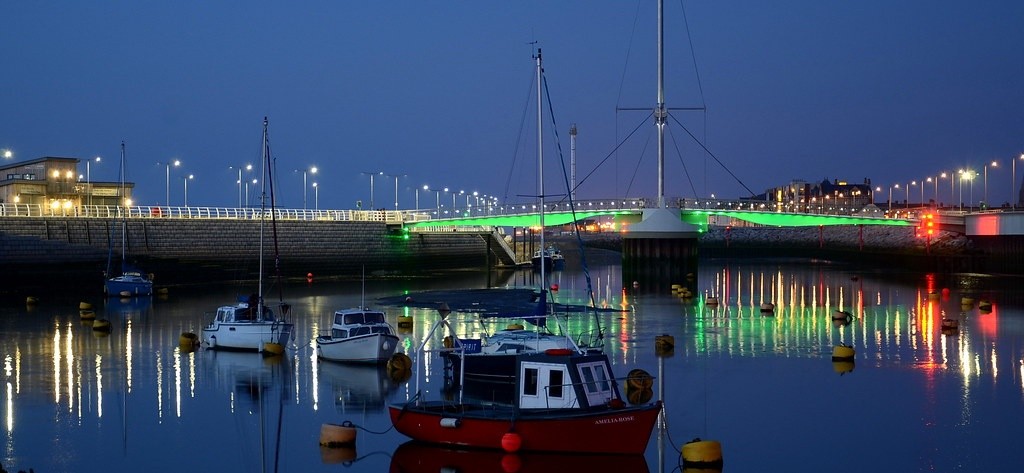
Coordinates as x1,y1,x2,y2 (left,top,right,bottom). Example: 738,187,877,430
103,140,156,298
202,117,296,355
386,41,668,456
383,438,655,473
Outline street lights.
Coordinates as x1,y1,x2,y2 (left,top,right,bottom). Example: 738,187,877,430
85,156,101,212
906,181,917,209
358,171,498,219
311,182,319,214
294,167,319,212
1012,153,1024,210
229,164,258,214
889,184,899,212
965,173,974,214
950,169,964,212
920,178,932,209
872,188,880,205
184,174,194,216
984,161,997,207
933,173,946,211
959,173,968,211
156,160,180,217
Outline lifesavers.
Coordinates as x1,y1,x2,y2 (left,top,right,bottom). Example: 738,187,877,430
545,349,574,355
149,273,155,281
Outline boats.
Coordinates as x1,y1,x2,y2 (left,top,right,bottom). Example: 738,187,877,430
546,246,566,266
316,264,400,363
532,245,553,266
